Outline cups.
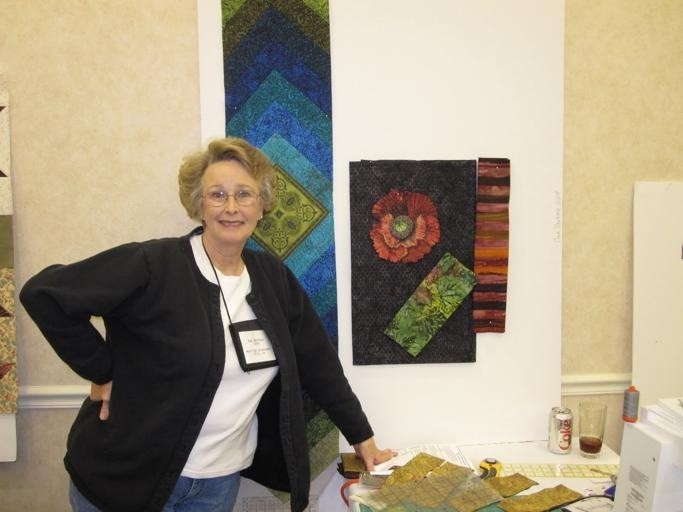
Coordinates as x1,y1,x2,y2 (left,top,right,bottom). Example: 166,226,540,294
577,400,607,459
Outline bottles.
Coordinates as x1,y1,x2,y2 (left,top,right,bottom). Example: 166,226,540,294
621,386,640,423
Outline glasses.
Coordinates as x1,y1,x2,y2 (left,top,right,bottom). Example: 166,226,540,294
198,189,260,206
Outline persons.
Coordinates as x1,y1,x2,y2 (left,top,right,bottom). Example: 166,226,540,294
19,136,401,512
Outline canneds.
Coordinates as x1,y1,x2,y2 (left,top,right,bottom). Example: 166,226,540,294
548,407,573,455
